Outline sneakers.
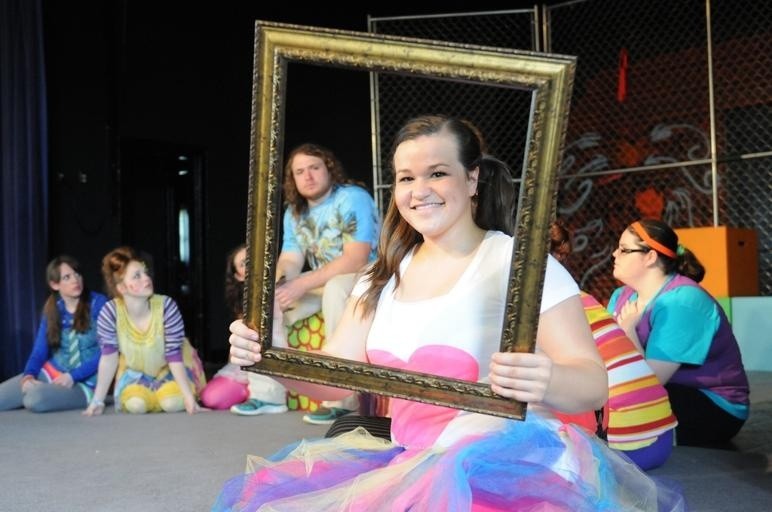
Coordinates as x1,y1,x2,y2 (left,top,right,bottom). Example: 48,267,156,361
231,397,288,416
303,406,360,425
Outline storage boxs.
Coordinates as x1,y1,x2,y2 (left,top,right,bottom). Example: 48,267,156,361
670,225,760,298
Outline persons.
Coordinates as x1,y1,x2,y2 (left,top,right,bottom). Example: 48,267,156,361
226,112,688,511
604,215,751,447
1,252,112,413
197,242,250,414
76,247,217,414
547,273,675,473
548,222,573,264
227,141,397,427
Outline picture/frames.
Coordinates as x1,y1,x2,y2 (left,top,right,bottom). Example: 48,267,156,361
238,17,579,423
